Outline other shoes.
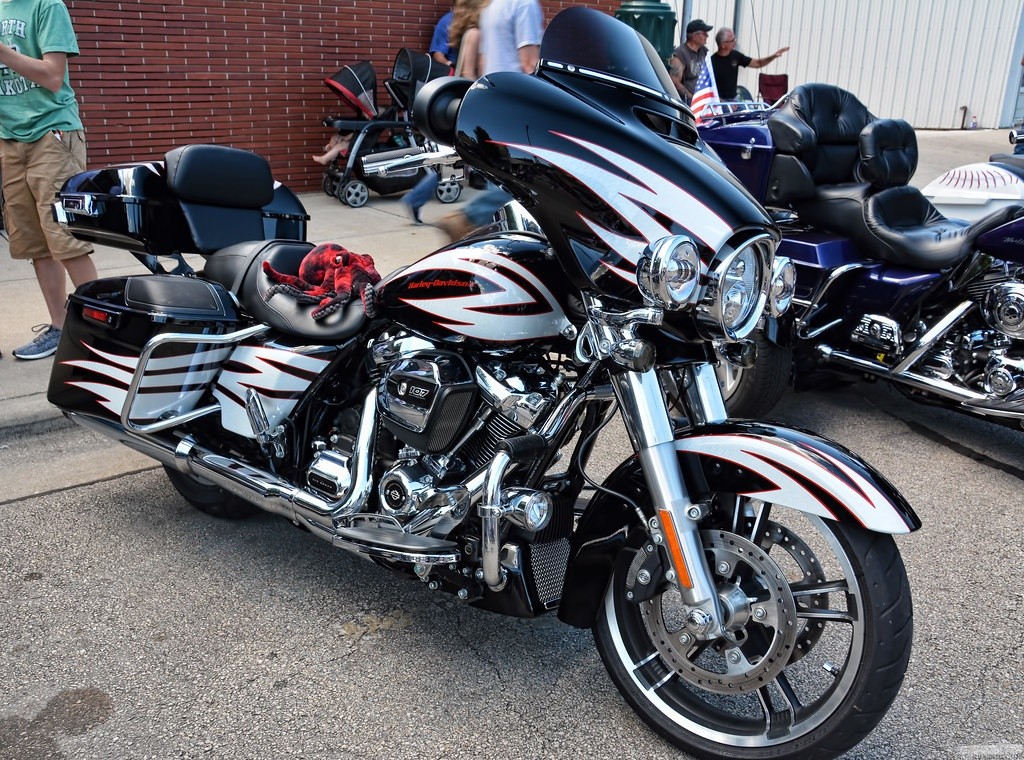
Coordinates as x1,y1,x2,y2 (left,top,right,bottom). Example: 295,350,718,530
408,204,424,224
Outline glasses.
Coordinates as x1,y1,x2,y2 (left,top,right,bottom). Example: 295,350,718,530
723,38,735,43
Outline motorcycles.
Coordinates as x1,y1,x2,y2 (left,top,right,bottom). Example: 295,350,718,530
491,78,1024,463
42,4,927,760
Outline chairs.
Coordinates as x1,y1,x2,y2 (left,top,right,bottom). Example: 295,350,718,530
768,83,878,237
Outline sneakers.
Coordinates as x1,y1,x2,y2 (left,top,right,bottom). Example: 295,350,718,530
12,324,62,360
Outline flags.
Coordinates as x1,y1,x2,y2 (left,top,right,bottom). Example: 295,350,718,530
691,55,718,128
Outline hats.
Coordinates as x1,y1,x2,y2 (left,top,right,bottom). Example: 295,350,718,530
687,20,713,33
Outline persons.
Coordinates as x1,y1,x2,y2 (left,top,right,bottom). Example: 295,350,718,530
0,0,99,359
438,0,543,243
670,19,714,106
429,0,488,190
710,27,790,101
398,0,497,226
313,105,408,166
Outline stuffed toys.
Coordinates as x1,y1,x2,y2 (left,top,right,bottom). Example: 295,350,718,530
262,243,382,320
325,134,346,152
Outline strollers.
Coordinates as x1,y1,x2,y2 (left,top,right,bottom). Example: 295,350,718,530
320,48,462,210
